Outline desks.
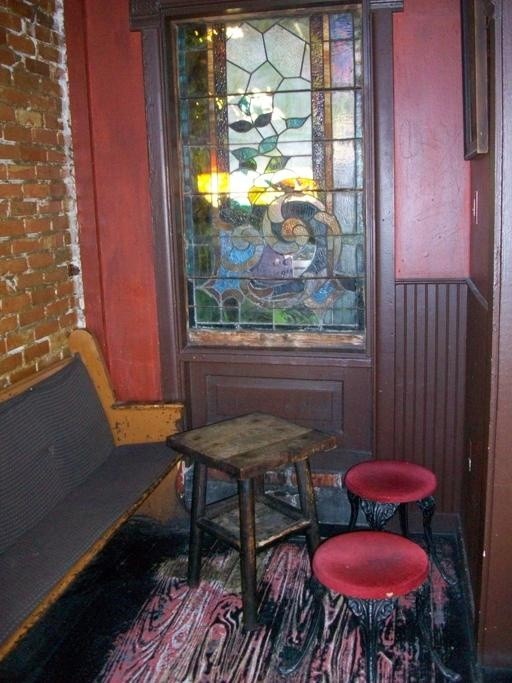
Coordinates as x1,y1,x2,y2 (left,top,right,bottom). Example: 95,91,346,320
167,412,337,631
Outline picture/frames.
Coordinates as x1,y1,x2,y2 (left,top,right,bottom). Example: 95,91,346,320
460,0,489,161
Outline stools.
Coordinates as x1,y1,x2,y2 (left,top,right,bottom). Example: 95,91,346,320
277,459,439,683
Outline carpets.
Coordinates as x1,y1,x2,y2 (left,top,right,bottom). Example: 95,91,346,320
36,521,474,683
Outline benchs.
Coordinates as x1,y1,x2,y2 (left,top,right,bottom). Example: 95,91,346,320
0,329,191,662
